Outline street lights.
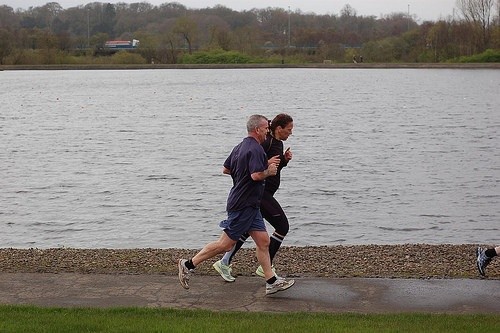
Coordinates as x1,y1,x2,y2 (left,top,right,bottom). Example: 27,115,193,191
288,5,291,47
87,9,90,48
407,4,410,32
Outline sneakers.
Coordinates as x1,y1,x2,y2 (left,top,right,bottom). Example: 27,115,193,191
178,258,194,289
256,264,287,280
213,260,236,282
266,279,295,295
476,246,492,275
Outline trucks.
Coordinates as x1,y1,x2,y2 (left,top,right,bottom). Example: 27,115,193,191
104,38,140,48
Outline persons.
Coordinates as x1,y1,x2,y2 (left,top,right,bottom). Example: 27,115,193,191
212,113,293,282
476,246,500,279
177,114,296,295
